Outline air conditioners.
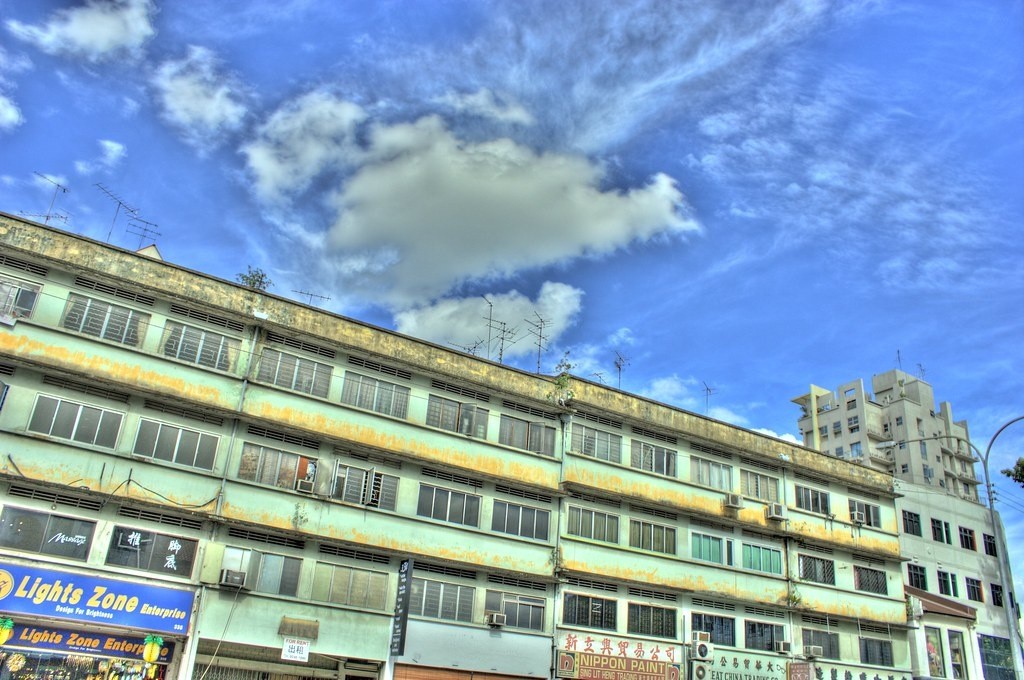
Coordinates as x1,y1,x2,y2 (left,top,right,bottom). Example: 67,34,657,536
687,660,712,680
803,645,823,658
724,493,743,509
767,502,788,521
690,641,714,661
219,568,247,588
693,631,710,643
775,641,790,654
850,511,866,525
488,613,506,626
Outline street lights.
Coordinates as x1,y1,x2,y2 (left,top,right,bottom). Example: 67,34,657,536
875,415,1024,680
